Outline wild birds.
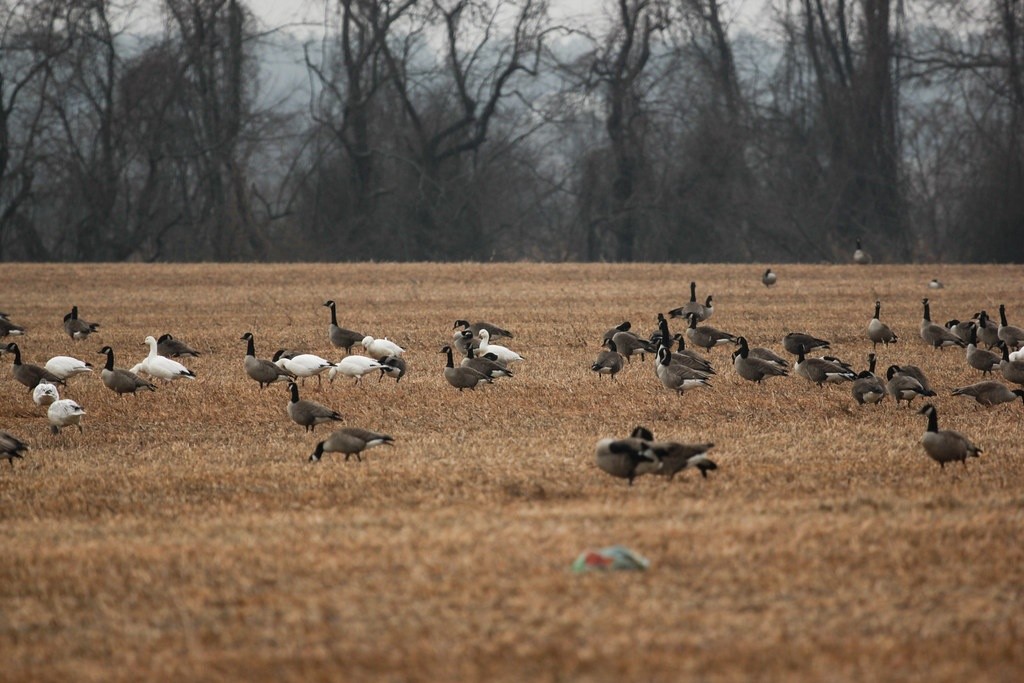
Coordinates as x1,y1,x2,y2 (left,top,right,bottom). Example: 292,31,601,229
4,342,93,437
853,234,873,266
284,384,346,433
0,312,27,342
761,266,777,284
243,332,408,389
61,307,100,341
916,406,983,471
157,336,205,364
308,425,396,463
0,431,26,465
595,427,719,486
592,279,1024,411
98,346,159,403
131,334,196,389
438,320,527,394
324,296,367,352
